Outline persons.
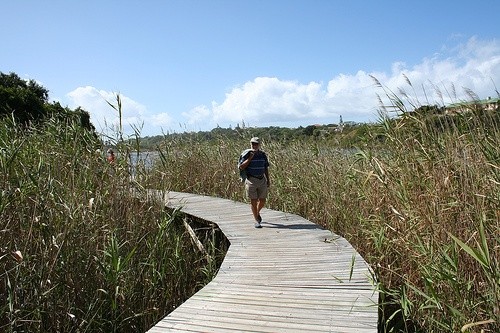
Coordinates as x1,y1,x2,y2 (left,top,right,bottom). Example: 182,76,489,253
238,137,270,228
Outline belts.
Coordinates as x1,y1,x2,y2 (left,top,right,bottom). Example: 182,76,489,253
246,174,264,180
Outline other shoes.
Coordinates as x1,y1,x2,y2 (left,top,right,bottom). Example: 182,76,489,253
258,215,261,222
255,222,261,228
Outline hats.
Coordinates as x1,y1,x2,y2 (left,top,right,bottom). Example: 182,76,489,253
250,137,262,144
